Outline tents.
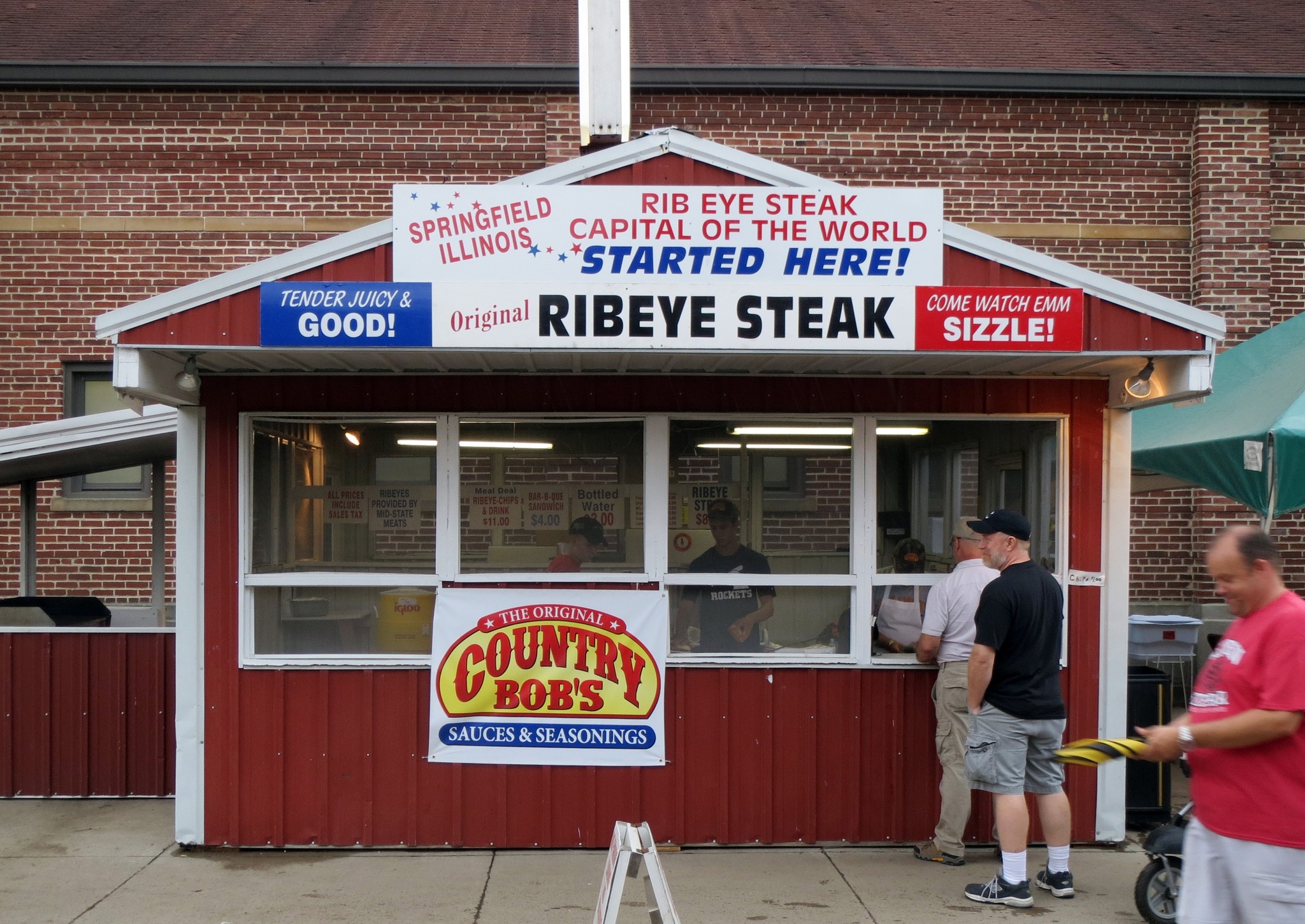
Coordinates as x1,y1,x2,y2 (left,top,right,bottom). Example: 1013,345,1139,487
1129,309,1305,539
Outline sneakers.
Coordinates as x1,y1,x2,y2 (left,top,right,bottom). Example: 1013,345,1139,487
1036,865,1074,897
913,840,965,865
964,868,1034,907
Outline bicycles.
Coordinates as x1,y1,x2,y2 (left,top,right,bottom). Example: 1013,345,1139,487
1134,751,1195,923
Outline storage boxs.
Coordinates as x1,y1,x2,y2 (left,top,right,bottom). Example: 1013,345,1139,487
1128,616,1204,663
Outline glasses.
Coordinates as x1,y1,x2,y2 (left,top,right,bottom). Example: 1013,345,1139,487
710,525,733,531
950,537,961,548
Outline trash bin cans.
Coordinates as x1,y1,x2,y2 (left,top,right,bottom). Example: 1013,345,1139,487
1126,665,1172,829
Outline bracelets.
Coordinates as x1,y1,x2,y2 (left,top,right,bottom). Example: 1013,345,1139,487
887,638,894,650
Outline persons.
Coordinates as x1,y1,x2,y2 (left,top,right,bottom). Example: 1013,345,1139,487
867,536,938,655
963,508,1078,909
912,513,1015,868
1131,520,1305,924
541,517,609,574
671,498,776,652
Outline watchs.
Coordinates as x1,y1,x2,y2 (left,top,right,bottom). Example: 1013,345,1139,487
1176,725,1194,752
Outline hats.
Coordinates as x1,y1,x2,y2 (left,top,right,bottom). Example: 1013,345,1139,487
947,516,984,544
569,517,609,548
707,499,741,522
894,537,926,575
966,509,1032,540
